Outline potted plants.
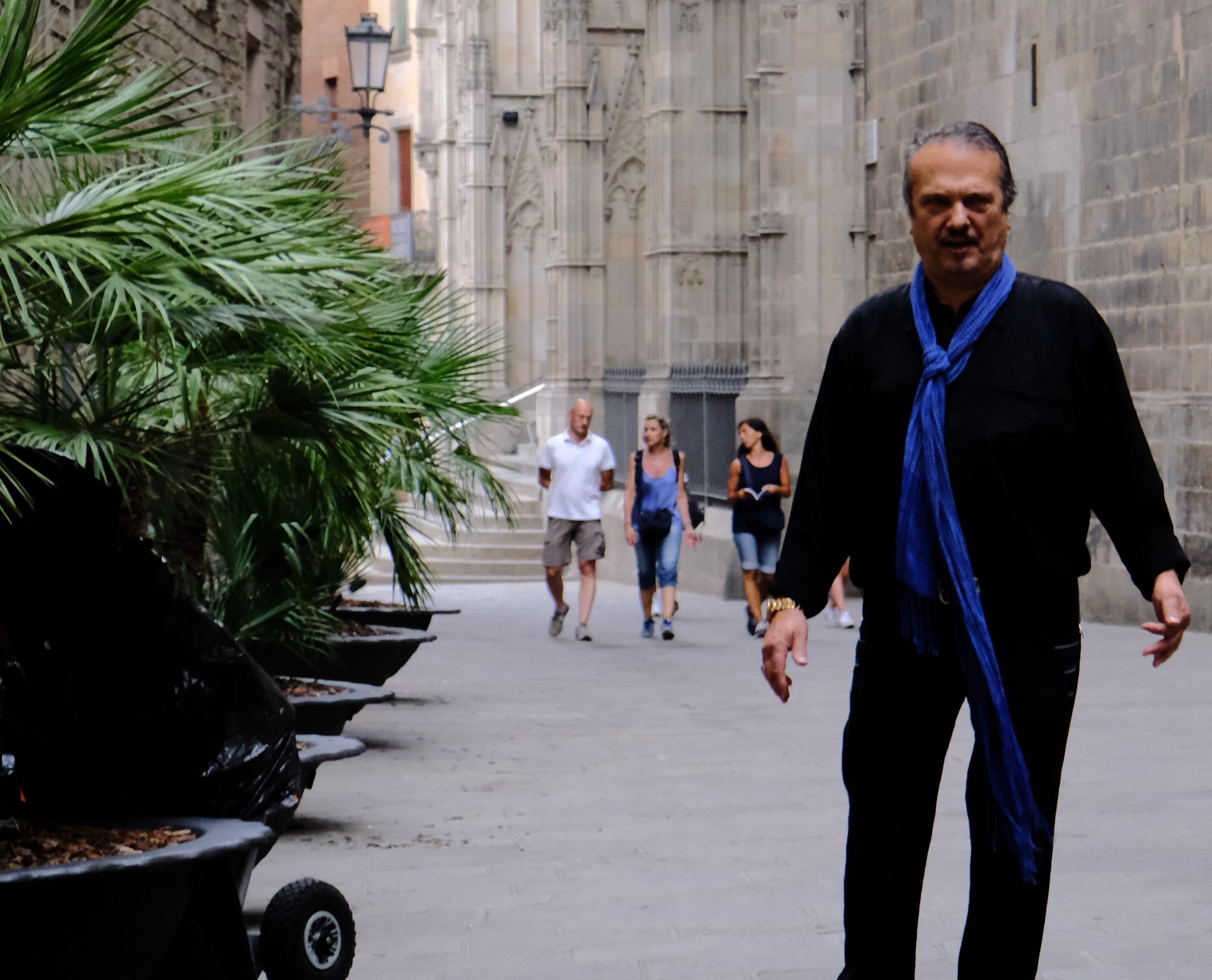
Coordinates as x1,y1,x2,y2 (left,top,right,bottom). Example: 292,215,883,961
0,0,527,980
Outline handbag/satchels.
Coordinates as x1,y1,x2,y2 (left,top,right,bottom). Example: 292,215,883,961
639,507,672,545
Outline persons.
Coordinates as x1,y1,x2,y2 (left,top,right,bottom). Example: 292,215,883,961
538,397,616,641
827,555,857,630
758,120,1191,980
624,414,702,639
727,416,790,638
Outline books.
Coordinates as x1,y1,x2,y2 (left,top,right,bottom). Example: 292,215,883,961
744,485,770,501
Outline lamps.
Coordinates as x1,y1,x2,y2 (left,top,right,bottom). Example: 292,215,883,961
280,10,394,146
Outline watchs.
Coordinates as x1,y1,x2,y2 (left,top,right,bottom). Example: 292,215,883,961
766,594,806,622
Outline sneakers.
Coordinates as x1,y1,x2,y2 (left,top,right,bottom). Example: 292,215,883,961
642,618,655,637
575,625,592,642
549,603,569,636
659,620,674,640
837,611,855,628
825,607,839,627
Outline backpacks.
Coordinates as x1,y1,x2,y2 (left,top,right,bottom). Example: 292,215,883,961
635,447,705,530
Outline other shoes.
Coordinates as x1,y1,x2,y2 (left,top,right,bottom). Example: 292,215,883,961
745,604,768,639
